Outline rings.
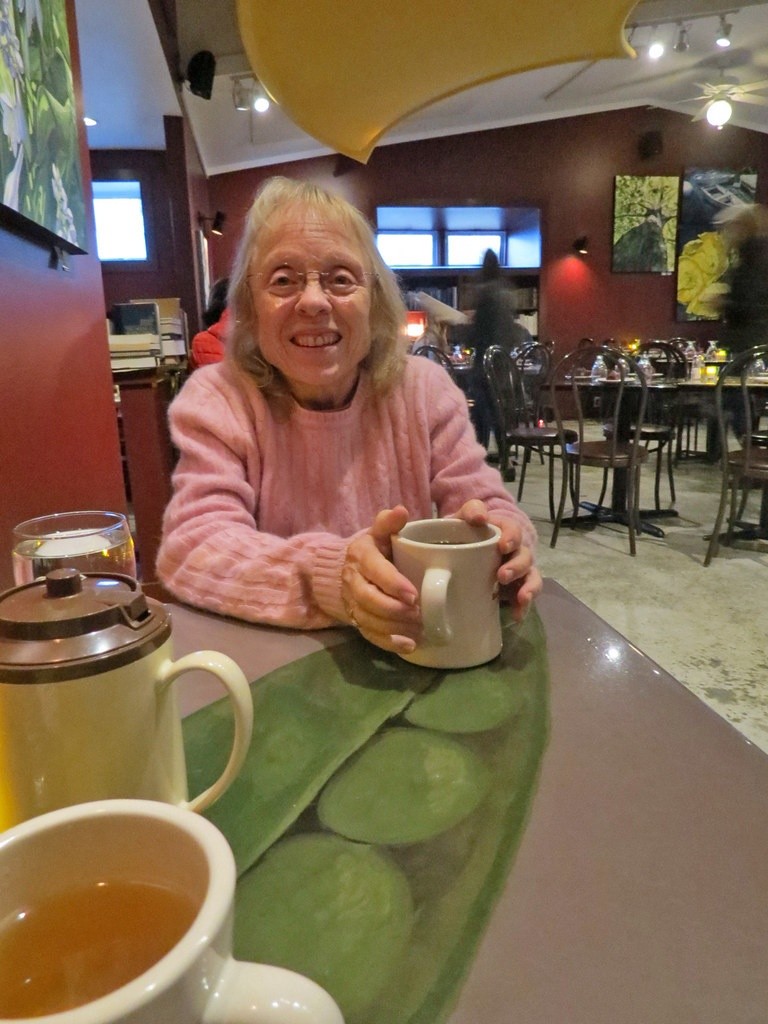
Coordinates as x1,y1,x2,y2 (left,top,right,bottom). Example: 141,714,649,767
349,610,359,628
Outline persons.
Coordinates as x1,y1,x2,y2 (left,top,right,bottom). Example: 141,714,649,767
155,175,542,653
466,289,533,451
190,277,226,365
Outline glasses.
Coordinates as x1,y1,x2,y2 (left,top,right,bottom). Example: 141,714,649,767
245,267,380,298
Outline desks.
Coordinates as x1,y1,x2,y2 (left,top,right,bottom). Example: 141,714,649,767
135,575,768,1024
591,348,731,428
676,376,768,542
539,375,680,539
430,356,545,468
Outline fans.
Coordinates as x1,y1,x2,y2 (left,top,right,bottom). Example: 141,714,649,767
645,67,768,131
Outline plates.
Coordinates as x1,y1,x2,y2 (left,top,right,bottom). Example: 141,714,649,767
595,377,635,386
748,373,768,384
565,376,600,383
627,373,664,379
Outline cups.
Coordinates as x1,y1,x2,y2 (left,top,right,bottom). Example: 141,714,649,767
692,357,701,380
391,518,502,669
13,510,137,586
0,799,345,1024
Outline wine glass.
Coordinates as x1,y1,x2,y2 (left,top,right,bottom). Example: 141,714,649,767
706,341,718,361
615,350,630,379
636,355,653,385
591,356,607,385
686,341,696,359
750,346,765,377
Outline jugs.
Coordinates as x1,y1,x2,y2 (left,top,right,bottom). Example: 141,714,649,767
0,568,253,837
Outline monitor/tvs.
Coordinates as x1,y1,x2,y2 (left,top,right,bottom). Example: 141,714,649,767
87,171,160,274
446,231,507,267
375,229,442,267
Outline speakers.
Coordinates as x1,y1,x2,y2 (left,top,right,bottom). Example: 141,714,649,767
186,51,216,101
643,132,663,156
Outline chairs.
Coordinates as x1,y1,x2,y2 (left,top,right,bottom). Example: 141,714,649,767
411,339,767,569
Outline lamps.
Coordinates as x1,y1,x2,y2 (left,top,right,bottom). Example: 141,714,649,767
232,79,250,110
671,23,691,53
716,13,734,47
707,97,735,129
572,237,589,256
196,210,227,236
